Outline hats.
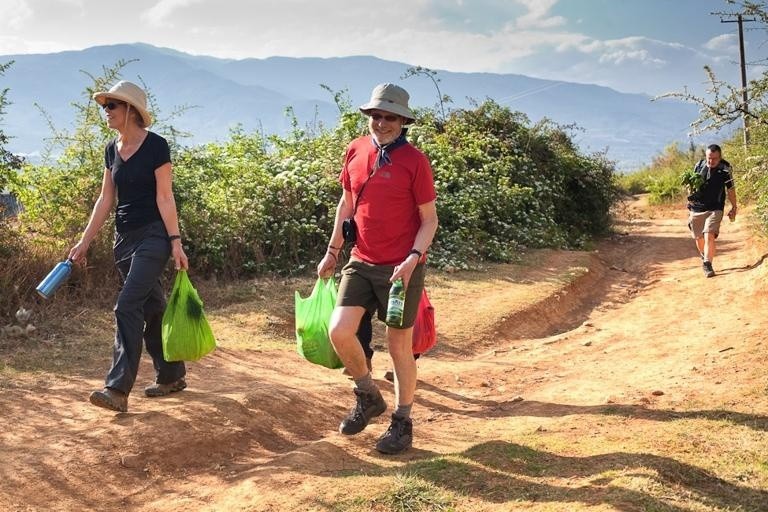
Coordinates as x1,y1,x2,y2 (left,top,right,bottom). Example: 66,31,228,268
92,80,151,128
358,83,416,125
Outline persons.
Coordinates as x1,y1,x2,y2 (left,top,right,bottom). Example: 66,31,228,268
687,144,735,278
69,80,189,413
314,82,438,455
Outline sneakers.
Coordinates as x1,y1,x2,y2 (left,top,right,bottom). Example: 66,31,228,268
703,261,716,278
90,389,128,414
144,379,187,397
376,413,414,454
341,387,386,435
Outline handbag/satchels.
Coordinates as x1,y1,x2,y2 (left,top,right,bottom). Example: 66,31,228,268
341,216,358,241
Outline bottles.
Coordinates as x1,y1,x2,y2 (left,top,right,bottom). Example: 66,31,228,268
34,257,73,300
384,274,406,329
728,210,735,224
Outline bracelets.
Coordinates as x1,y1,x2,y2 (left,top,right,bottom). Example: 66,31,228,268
328,251,337,263
165,232,181,242
409,247,422,257
328,245,340,250
